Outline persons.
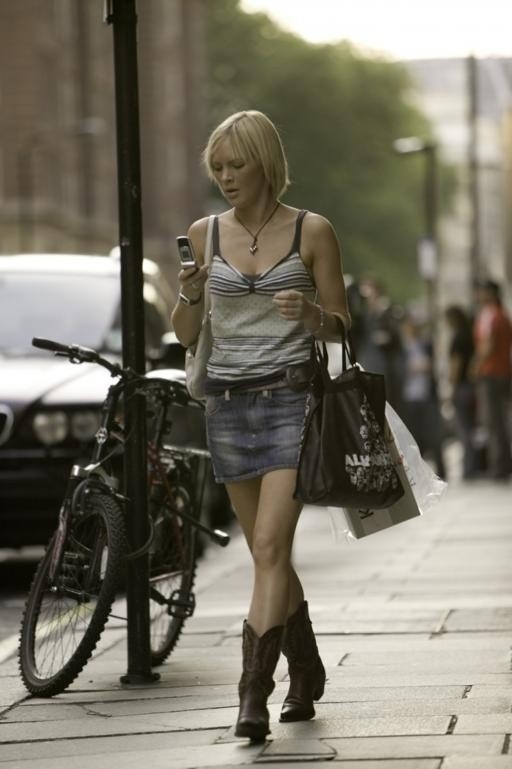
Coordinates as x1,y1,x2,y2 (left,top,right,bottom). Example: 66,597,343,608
345,272,510,481
171,110,354,741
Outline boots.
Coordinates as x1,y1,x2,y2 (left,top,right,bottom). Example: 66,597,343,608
235,617,285,745
279,598,327,724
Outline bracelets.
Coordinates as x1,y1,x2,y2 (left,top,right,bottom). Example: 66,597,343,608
179,289,204,305
312,301,325,340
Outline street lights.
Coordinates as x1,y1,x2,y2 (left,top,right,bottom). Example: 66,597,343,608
392,135,446,482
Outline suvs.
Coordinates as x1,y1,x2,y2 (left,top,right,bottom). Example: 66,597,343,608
1,245,237,576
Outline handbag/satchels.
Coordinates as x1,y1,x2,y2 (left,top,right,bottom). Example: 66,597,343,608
290,312,407,512
315,311,421,541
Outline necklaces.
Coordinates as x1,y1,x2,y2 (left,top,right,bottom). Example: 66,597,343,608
232,201,280,254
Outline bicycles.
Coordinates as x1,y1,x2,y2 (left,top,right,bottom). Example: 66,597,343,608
18,335,230,699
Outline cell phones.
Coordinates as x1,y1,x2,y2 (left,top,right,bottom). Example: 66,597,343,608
176,236,199,277
